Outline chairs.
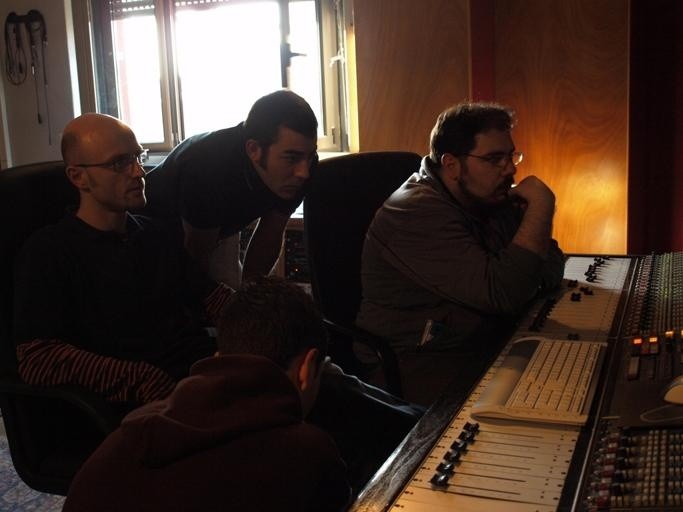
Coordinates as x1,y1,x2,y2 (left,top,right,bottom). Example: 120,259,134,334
0,158,111,497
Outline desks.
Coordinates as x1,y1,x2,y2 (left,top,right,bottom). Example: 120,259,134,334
348,251,683,512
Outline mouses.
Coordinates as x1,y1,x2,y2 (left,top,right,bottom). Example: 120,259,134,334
662,375,683,404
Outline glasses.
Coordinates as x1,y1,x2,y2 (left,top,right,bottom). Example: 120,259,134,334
75,149,149,173
456,151,523,167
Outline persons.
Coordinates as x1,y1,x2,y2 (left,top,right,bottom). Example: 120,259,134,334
141,88,320,325
12,111,236,402
63,275,355,507
353,102,562,406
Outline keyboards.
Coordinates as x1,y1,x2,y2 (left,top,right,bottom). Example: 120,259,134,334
471,335,609,423
